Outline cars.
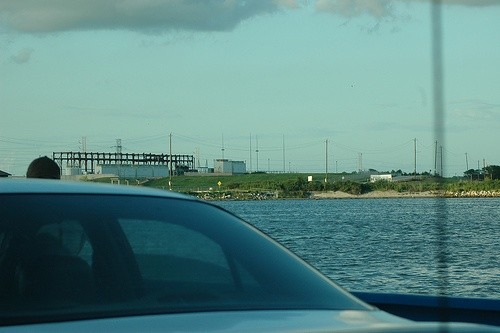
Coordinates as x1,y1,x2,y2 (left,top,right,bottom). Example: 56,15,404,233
0,178,496,333
